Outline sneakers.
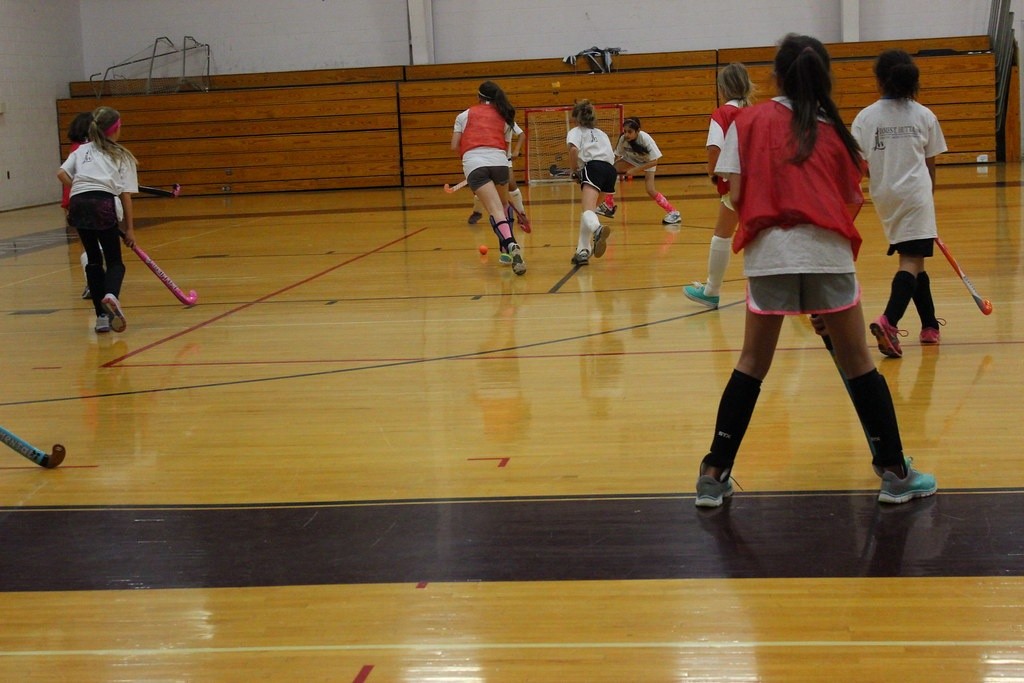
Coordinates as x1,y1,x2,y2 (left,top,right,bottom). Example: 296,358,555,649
570,250,590,268
593,225,611,257
662,212,682,225
694,467,744,507
468,211,483,226
683,281,719,309
499,253,511,265
595,201,619,219
95,317,111,333
517,214,531,234
102,293,126,332
871,455,938,504
870,316,910,358
507,243,526,275
919,316,946,343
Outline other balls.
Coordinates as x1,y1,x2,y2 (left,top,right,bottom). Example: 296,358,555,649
480,244,490,256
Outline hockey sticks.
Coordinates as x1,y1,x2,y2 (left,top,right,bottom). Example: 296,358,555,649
0,424,67,468
570,172,618,218
935,237,994,316
137,181,183,199
443,151,522,195
548,164,628,179
113,226,198,306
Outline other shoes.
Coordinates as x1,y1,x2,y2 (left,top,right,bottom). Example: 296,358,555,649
81,287,90,300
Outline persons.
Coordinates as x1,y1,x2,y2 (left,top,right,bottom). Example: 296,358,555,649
851,48,950,356
595,116,682,223
694,33,938,509
450,82,533,275
565,98,617,265
55,109,140,336
468,121,531,233
681,64,758,311
